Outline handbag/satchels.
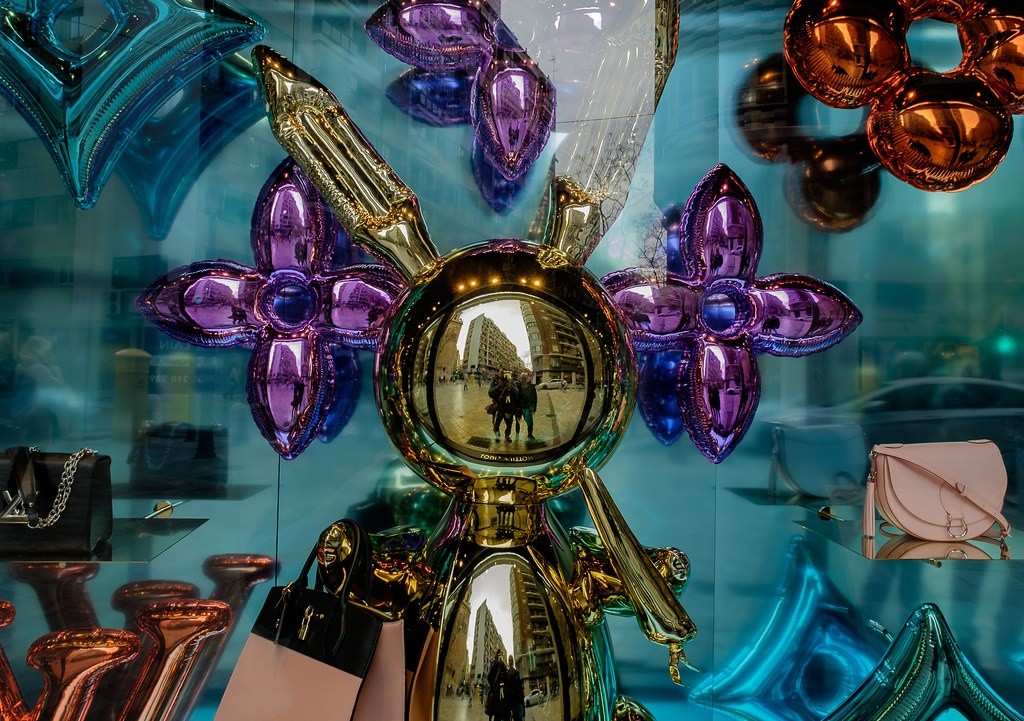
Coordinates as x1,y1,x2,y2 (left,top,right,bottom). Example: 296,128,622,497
862,439,1012,541
485,403,498,413
213,517,405,721
0,445,113,553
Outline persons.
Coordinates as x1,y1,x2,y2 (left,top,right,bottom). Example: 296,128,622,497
561,378,568,391
469,685,474,707
365,305,384,326
508,122,520,150
294,237,308,267
226,304,248,324
710,248,723,276
707,380,723,430
464,378,469,391
624,307,651,330
488,369,538,442
289,377,305,417
485,650,526,721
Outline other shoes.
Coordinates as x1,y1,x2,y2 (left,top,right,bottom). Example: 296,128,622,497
493,428,501,438
527,434,536,440
505,435,512,443
